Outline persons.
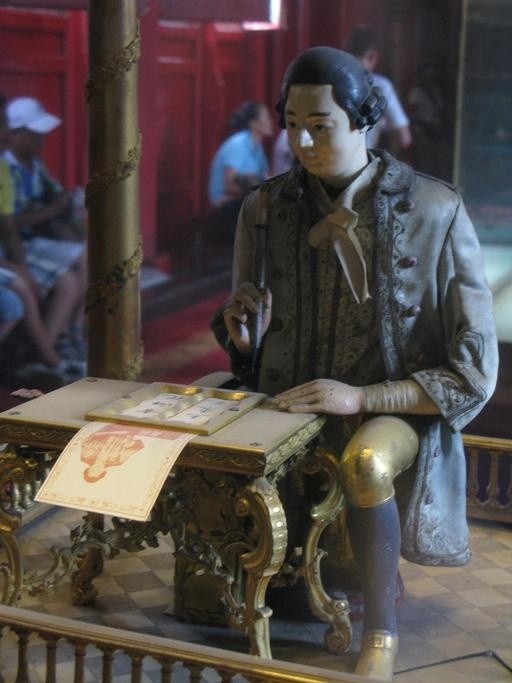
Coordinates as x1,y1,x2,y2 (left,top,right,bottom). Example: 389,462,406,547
406,61,450,176
208,46,500,681
78,419,188,485
272,128,296,176
3,97,88,360
347,26,412,158
0,153,72,389
207,101,274,223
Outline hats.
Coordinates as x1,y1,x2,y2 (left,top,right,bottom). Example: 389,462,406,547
4,97,63,135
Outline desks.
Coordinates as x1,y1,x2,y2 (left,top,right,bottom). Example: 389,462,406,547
0,373,355,683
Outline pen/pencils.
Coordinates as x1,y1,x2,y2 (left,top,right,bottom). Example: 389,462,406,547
251,183,271,348
